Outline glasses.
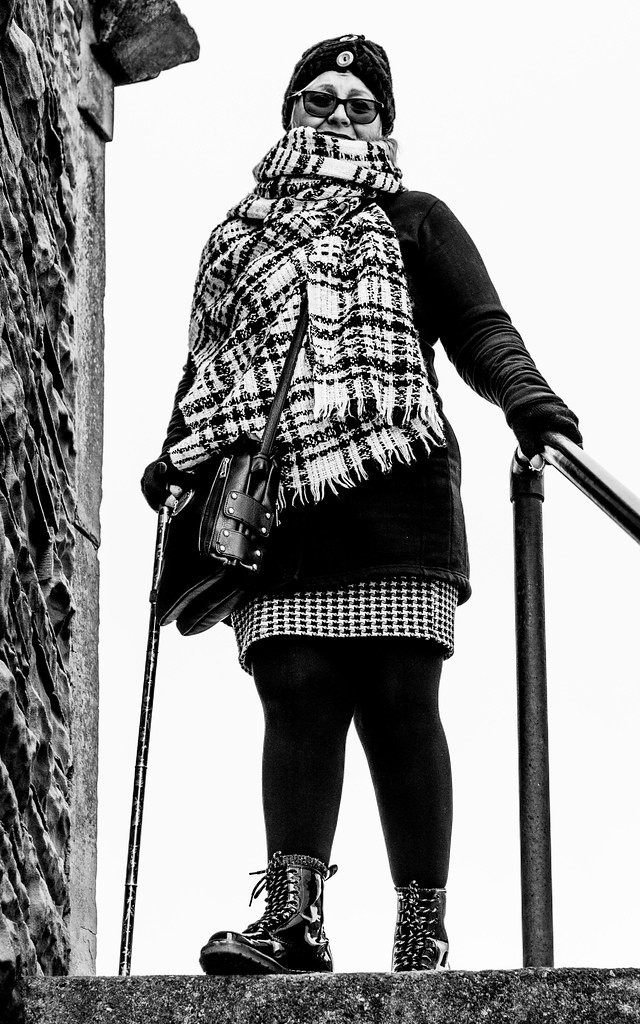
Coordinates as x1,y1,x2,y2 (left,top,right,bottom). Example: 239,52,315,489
289,87,385,125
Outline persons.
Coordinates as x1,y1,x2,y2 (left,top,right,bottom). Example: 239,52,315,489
140,36,582,974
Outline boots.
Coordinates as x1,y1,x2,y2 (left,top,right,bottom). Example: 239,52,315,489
391,889,448,972
199,852,338,974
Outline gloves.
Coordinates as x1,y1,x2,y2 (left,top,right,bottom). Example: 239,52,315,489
142,456,196,513
509,399,583,470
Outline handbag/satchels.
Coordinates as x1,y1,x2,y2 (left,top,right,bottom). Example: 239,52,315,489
153,454,281,635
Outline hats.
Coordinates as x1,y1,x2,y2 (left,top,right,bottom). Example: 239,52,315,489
282,35,397,136
